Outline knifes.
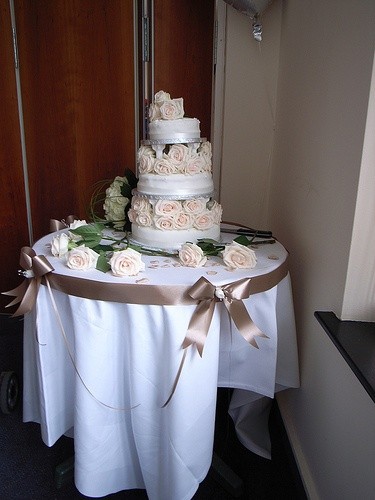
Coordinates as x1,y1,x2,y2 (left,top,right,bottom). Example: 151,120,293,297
220,227,272,237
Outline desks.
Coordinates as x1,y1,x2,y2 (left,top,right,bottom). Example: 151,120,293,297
19,214,304,500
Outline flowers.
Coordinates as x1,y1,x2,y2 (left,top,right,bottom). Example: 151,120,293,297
101,165,138,228
126,91,224,231
110,230,146,278
45,232,143,258
221,230,260,272
64,229,133,269
177,237,276,269
68,218,142,252
